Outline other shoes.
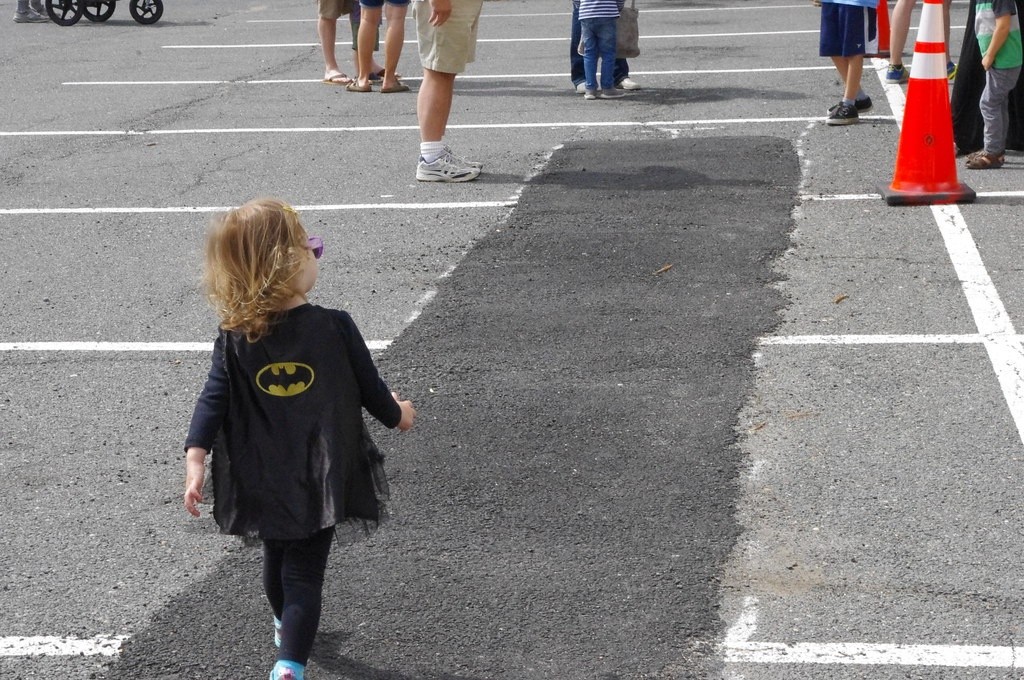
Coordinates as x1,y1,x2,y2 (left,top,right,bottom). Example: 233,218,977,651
616,78,639,89
576,83,586,95
275,624,282,647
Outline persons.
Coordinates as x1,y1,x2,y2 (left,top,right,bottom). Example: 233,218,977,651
181,200,416,680
410,0,485,184
814,0,878,125
885,0,958,84
318,0,414,93
966,0,1022,169
569,0,642,100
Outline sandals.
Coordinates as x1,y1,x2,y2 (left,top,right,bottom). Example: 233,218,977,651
965,148,1005,168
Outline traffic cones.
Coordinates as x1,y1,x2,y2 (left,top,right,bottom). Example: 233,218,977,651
875,0,978,203
864,0,898,57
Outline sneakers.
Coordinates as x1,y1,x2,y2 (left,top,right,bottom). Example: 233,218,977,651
38,8,61,21
886,64,910,84
417,145,482,183
826,102,859,126
600,87,625,98
947,61,957,81
13,10,47,23
584,88,598,99
827,94,873,116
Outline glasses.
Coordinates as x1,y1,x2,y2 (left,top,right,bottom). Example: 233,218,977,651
299,237,324,259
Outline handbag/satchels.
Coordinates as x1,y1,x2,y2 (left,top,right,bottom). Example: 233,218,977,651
578,0,640,59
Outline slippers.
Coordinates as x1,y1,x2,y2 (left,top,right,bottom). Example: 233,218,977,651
379,80,408,93
355,65,402,80
345,80,371,92
323,69,352,85
